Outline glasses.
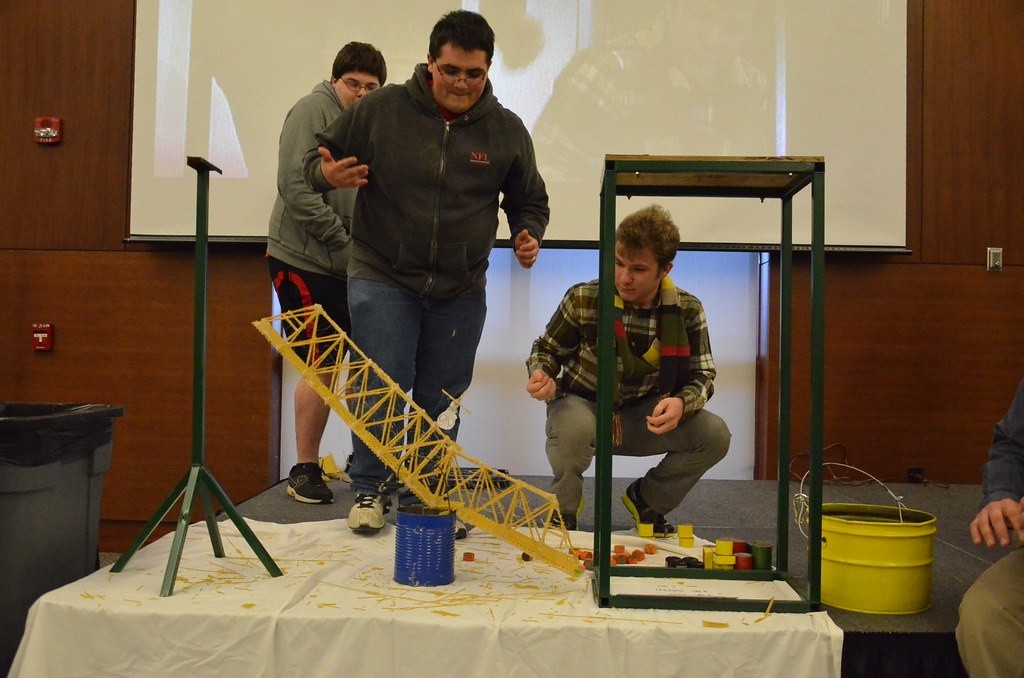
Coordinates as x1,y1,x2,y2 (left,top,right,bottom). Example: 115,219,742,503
433,57,488,84
339,75,379,93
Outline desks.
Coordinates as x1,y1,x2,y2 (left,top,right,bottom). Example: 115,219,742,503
592,154,827,615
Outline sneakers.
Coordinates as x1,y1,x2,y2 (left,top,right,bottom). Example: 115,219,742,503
549,494,585,531
454,520,467,539
621,477,675,538
343,455,405,488
348,494,386,529
287,462,335,503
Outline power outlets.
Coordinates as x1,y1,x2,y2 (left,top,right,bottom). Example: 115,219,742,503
987,247,1003,271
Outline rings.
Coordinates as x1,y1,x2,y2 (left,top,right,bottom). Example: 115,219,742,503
533,256,537,262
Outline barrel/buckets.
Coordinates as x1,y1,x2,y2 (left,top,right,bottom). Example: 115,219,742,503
798,462,936,614
394,455,457,588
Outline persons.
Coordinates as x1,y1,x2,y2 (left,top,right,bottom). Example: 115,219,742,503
266,41,407,505
525,204,731,536
955,382,1024,678
300,11,551,537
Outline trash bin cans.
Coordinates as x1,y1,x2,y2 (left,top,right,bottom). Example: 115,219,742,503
0,401,123,678
